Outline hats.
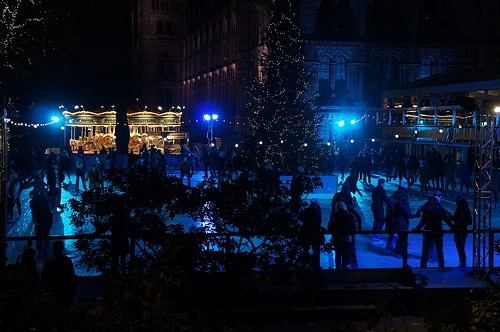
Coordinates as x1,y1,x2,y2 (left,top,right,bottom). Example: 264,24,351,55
311,198,320,206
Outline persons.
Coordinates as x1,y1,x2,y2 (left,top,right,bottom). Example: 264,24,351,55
0,141,235,306
294,146,473,268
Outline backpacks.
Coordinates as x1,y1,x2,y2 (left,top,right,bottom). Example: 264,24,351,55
333,213,352,243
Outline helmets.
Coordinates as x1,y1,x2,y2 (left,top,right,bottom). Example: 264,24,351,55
335,201,347,210
